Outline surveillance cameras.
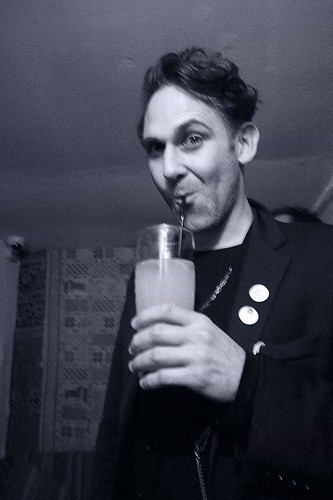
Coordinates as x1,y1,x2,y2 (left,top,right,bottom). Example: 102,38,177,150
6,236,27,253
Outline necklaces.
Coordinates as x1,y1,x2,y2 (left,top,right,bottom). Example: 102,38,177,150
194,265,232,314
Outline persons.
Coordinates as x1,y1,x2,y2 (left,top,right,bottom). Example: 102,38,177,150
93,47,331,500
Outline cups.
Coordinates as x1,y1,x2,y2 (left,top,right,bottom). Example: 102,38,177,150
134,225,197,328
